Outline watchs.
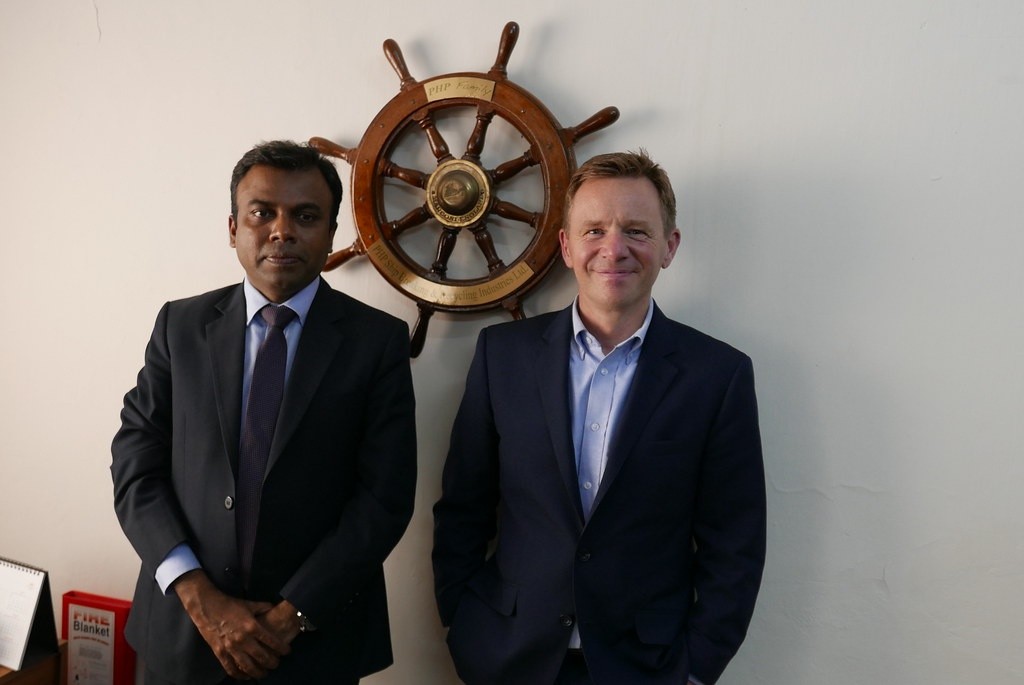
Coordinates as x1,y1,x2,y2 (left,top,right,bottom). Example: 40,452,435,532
296,610,317,632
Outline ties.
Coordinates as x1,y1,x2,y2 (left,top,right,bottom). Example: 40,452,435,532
236,304,299,582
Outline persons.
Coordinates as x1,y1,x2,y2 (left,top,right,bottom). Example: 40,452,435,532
432,147,768,685
110,139,418,685
